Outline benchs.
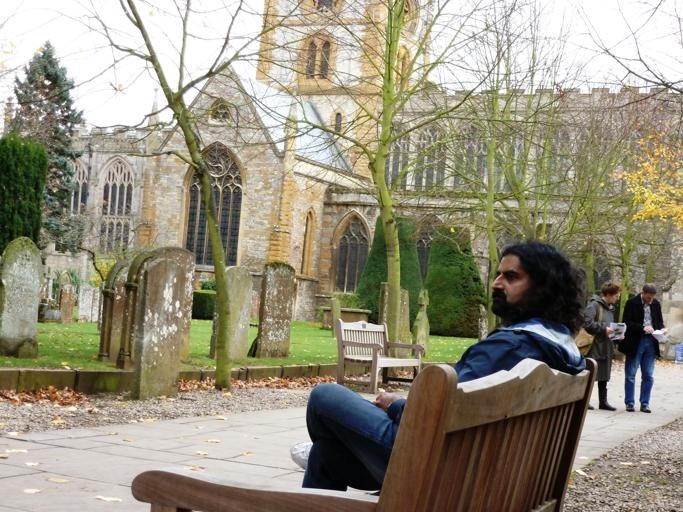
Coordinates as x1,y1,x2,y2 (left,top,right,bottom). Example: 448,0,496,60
130,320,599,511
334,320,426,394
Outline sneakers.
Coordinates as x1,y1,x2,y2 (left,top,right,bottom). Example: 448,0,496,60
627,405,635,411
641,406,652,413
290,441,314,470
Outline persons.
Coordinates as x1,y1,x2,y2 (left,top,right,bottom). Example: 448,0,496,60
290,241,585,491
583,283,625,410
617,284,667,412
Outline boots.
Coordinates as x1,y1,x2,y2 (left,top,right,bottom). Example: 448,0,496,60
599,389,616,411
589,405,595,409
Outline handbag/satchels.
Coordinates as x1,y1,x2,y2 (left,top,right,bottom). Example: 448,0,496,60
574,328,595,356
